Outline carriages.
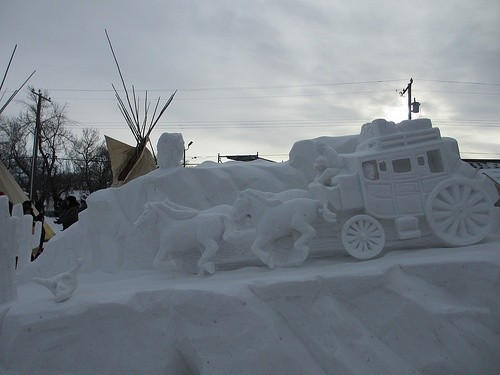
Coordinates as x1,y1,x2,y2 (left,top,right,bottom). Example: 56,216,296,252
130,125,495,277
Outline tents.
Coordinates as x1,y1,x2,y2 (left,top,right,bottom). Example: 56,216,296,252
0,160,58,243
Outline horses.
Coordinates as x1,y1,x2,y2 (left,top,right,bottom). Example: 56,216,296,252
129,182,339,277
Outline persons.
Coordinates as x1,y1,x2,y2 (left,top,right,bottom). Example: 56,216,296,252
0,189,92,268
308,139,344,189
